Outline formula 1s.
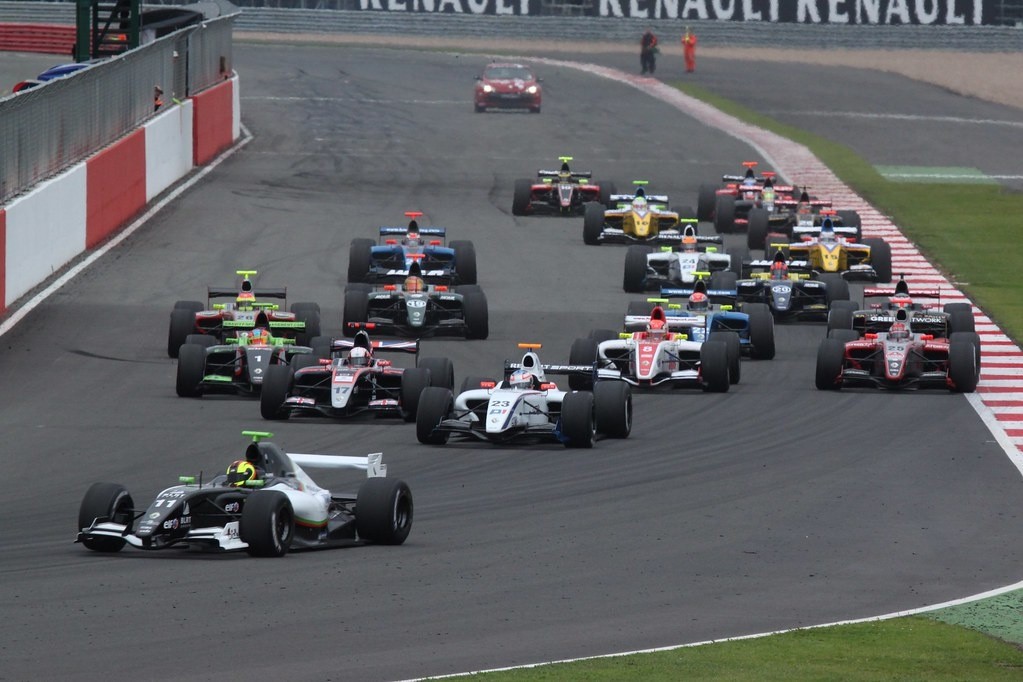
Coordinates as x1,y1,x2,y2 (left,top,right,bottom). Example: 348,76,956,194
343,252,488,340
343,210,477,287
259,318,453,428
416,340,633,451
755,209,895,282
811,287,983,396
821,270,979,345
690,161,862,247
566,299,741,393
75,430,417,558
619,218,740,301
621,269,778,360
512,154,617,218
176,302,329,398
584,178,699,245
725,244,852,320
161,269,321,361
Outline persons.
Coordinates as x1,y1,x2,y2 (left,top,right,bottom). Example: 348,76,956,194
637,27,660,76
558,170,572,182
217,458,257,487
761,187,776,202
402,232,422,246
509,370,534,391
153,86,163,113
71,41,77,61
235,292,256,312
347,347,372,368
248,328,272,347
889,323,910,339
631,196,648,214
689,292,708,312
890,292,914,309
646,319,667,340
680,26,697,75
681,236,698,252
795,201,812,214
402,275,429,294
818,229,837,242
741,176,758,185
770,261,790,281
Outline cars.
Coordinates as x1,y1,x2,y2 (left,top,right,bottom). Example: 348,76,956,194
14,61,94,95
473,60,543,112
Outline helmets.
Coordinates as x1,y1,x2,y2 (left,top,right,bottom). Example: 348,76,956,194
770,261,788,280
680,237,698,252
227,461,259,489
404,275,426,294
818,231,835,241
646,320,668,340
891,293,913,311
889,324,910,340
742,177,758,187
761,187,776,200
405,232,421,246
508,369,535,391
558,171,572,183
237,293,256,312
688,293,708,311
246,330,273,346
632,196,648,209
796,201,812,213
347,348,373,368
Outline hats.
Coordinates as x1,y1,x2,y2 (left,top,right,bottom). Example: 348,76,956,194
155,85,163,94
